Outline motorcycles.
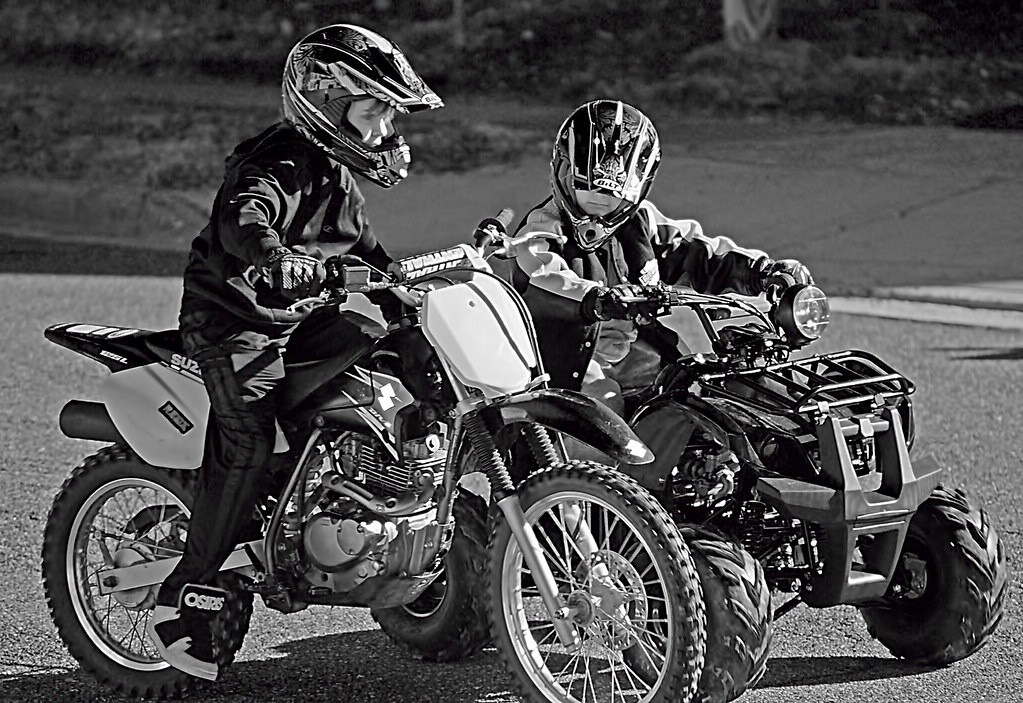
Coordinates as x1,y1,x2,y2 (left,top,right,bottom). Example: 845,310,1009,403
370,271,1012,703
44,208,707,703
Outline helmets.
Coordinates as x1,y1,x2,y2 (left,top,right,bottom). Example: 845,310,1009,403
281,23,446,189
549,99,662,252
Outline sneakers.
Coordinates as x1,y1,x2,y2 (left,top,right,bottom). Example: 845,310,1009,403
146,582,229,682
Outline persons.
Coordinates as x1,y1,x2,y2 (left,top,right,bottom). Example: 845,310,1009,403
146,19,444,682
508,99,813,457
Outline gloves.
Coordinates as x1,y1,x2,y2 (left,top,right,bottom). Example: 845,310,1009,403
263,246,325,300
748,255,815,292
584,284,650,319
